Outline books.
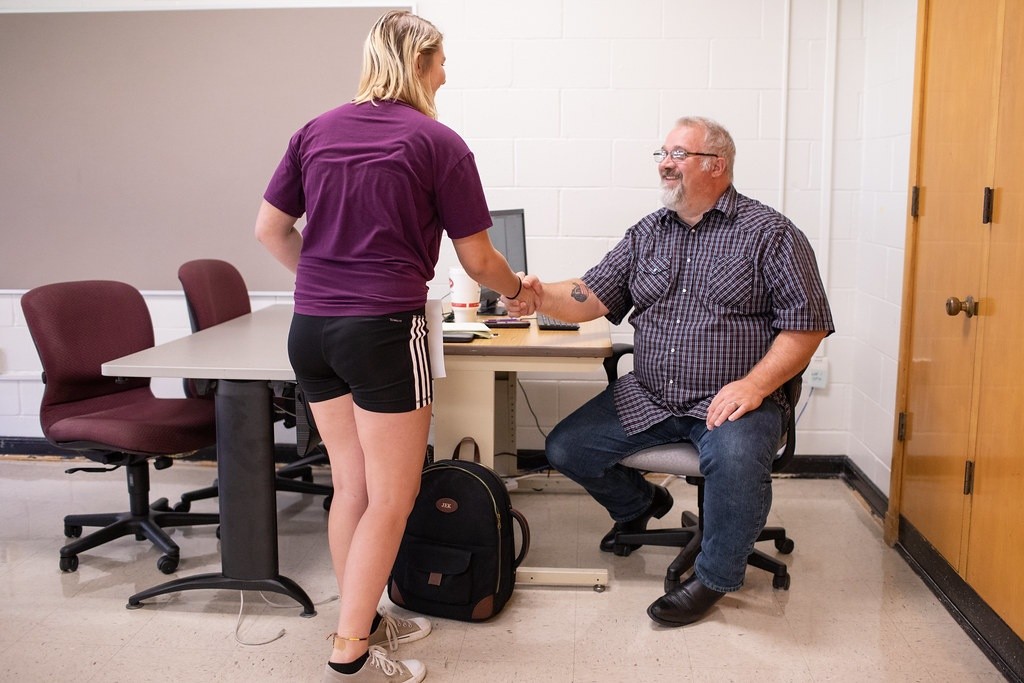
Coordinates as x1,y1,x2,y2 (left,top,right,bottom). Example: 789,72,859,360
442,322,492,332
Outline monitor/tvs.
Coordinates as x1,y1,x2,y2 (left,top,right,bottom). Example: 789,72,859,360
477,208,528,316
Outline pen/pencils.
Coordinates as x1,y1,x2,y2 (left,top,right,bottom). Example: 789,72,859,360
482,319,530,323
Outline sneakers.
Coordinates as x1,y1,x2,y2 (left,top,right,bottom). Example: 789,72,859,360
368,606,432,648
323,646,427,683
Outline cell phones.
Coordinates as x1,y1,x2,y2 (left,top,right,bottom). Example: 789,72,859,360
485,322,530,328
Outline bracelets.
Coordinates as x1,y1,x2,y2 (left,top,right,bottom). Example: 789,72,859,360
505,276,522,299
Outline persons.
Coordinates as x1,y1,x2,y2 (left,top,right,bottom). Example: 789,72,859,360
254,11,541,683
502,115,835,628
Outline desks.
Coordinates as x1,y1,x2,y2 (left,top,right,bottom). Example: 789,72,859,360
432,301,618,596
98,301,319,618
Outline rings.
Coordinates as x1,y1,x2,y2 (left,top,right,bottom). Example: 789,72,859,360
731,402,738,407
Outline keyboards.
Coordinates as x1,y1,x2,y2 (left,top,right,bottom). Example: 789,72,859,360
536,311,580,331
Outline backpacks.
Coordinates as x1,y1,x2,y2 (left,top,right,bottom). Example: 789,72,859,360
387,436,530,621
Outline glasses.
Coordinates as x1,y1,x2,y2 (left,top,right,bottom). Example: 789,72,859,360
653,147,719,163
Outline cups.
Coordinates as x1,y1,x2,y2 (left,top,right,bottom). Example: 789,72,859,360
448,265,480,323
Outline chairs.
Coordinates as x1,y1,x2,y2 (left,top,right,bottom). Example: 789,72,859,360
599,340,810,594
173,256,332,512
18,278,215,572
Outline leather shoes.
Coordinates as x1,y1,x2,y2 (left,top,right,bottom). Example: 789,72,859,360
600,485,674,552
647,571,726,627
